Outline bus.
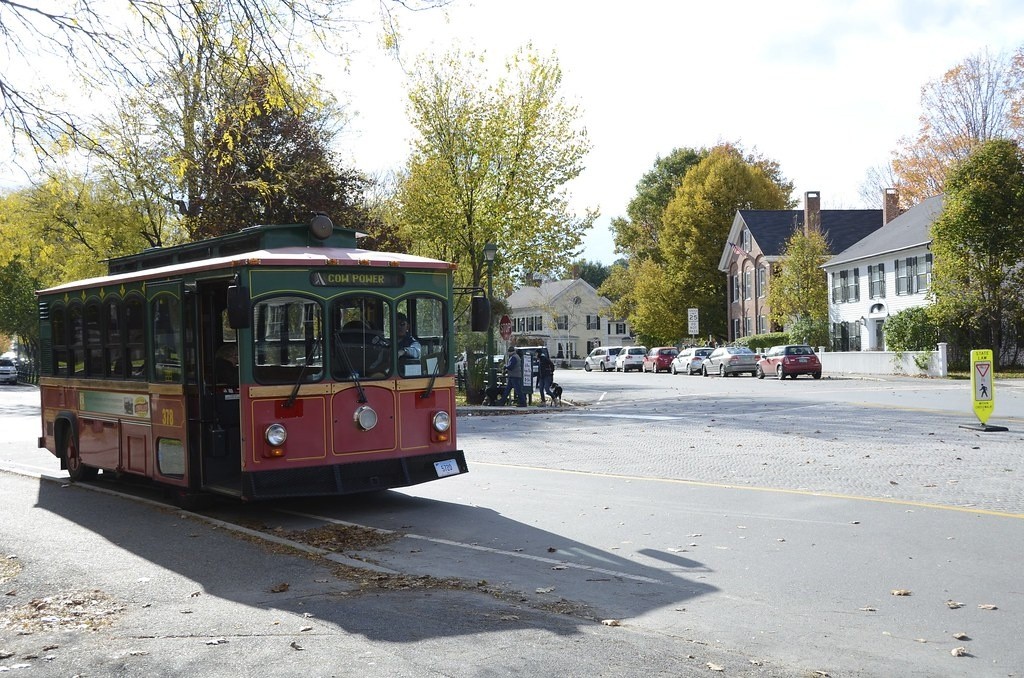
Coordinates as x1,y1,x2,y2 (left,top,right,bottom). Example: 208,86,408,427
34,212,468,511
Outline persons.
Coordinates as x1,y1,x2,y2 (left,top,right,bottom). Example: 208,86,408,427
213,344,238,384
369,313,421,374
534,349,559,408
495,346,527,407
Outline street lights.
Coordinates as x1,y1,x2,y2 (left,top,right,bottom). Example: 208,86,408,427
482,244,499,406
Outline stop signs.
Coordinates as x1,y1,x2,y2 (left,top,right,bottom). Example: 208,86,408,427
500,315,511,340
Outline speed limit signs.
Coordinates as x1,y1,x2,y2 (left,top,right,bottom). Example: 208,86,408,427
688,309,698,321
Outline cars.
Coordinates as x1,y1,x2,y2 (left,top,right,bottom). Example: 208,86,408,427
671,347,715,376
702,348,760,377
477,355,506,381
755,345,822,380
615,346,649,372
498,346,550,382
0,359,18,385
455,351,487,375
642,347,680,374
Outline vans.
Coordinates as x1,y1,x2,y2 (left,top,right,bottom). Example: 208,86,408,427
584,347,624,372
